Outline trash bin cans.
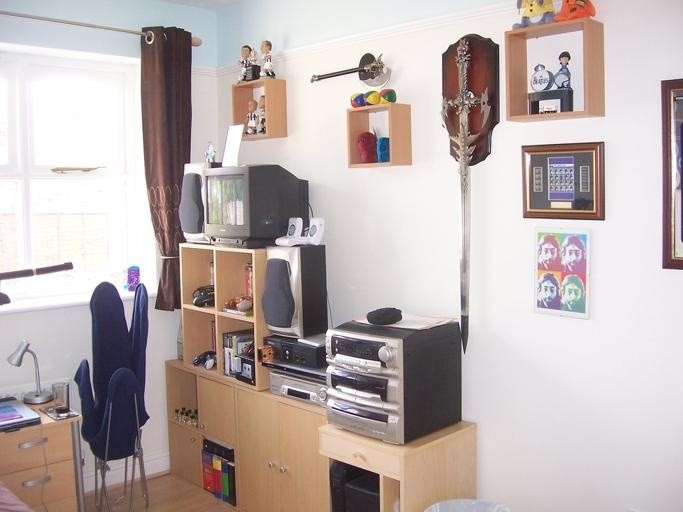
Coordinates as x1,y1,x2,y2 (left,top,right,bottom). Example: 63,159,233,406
425,498,510,512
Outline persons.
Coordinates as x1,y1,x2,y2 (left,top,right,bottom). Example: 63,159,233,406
245,100,260,134
236,45,259,84
259,95,266,135
554,51,571,89
260,40,276,78
536,231,586,314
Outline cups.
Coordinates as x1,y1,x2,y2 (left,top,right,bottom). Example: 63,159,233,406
51,382,69,417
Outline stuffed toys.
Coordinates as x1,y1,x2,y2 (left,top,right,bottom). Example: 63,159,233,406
512,0,558,30
554,0,595,22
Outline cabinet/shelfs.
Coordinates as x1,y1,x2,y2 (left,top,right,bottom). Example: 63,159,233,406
0,400,82,512
179,242,270,392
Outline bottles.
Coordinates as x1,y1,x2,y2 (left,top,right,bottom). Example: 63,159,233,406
242,261,253,300
170,407,200,428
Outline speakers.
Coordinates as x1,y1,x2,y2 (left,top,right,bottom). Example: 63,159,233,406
178,162,222,244
262,245,328,338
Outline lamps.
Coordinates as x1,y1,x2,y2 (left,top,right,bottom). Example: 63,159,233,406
7,340,54,404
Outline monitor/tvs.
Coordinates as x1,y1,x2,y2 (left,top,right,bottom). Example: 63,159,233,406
207,163,310,247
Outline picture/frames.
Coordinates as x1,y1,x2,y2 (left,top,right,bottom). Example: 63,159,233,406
661,79,683,270
531,224,590,320
521,141,605,220
